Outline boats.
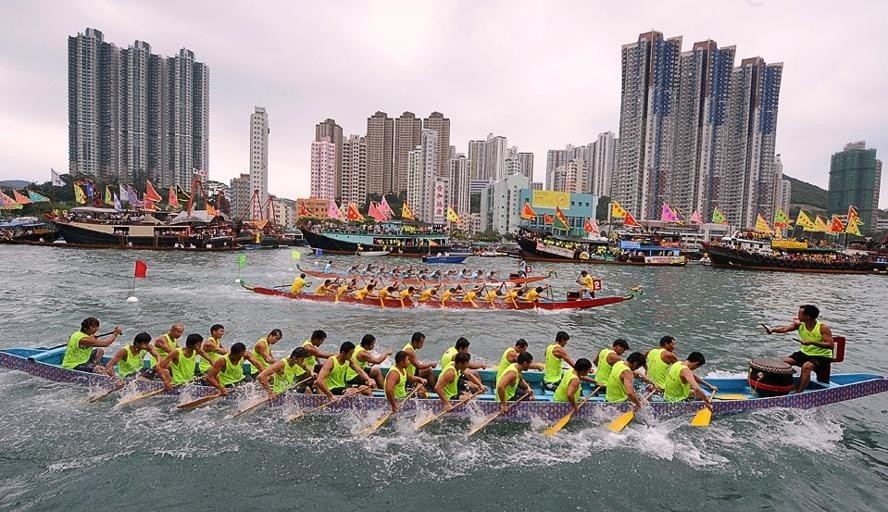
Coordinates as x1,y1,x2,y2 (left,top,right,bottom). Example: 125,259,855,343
512,225,689,267
474,248,509,258
700,230,888,275
240,279,643,311
427,256,471,264
0,216,63,246
480,250,508,257
450,248,474,256
0,347,884,416
0,336,888,423
298,219,475,258
422,256,467,264
240,286,635,310
44,205,241,252
699,257,711,266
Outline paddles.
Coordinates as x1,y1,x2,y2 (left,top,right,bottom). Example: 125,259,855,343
87,365,152,405
440,281,445,307
484,283,495,310
289,384,373,423
35,326,123,350
177,386,237,410
116,376,203,406
376,280,385,311
417,388,485,429
364,381,425,437
395,281,407,309
459,285,478,308
544,384,602,436
469,390,534,437
690,388,716,428
232,375,316,418
607,388,657,433
503,282,519,309
333,291,338,305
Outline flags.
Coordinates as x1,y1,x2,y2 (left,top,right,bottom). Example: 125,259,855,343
146,180,162,202
75,184,85,204
86,181,94,197
168,185,181,207
831,214,844,234
119,182,129,201
674,207,686,223
522,201,537,221
238,255,250,268
327,199,346,222
402,202,415,220
556,206,571,226
367,200,378,218
844,216,862,237
446,207,461,223
300,201,315,218
128,184,137,204
827,218,838,236
26,188,51,203
711,206,727,224
803,226,815,232
775,217,793,231
545,212,554,224
134,260,148,279
176,185,190,202
290,250,305,260
51,168,67,187
104,186,113,203
814,215,826,233
13,188,32,204
206,204,217,215
623,211,643,229
347,203,364,223
114,192,121,209
611,200,628,218
381,196,395,215
690,210,704,224
144,192,156,210
754,213,775,234
795,209,815,227
776,207,795,225
129,198,141,207
846,204,864,227
373,206,386,220
553,215,569,230
585,220,598,233
660,201,679,225
0,189,23,209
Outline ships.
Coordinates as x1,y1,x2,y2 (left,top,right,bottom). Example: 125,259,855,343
725,234,846,254
0,214,57,250
704,241,887,281
520,234,687,269
297,223,454,253
45,213,236,250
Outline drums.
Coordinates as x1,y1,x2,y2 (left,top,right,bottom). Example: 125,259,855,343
747,357,797,397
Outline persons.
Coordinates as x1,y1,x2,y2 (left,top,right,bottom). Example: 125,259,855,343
434,284,438,287
0,207,284,252
494,285,500,289
331,278,345,290
463,284,485,302
302,330,337,371
399,285,415,304
290,273,312,294
154,322,184,363
351,278,364,292
495,338,545,383
761,305,834,394
485,280,512,300
576,271,595,301
440,287,456,302
357,285,379,300
250,329,282,378
300,220,513,280
257,347,318,401
205,343,265,395
418,284,442,302
593,339,625,394
345,334,393,390
60,317,122,375
107,332,162,387
606,351,654,413
338,284,354,292
551,358,600,412
313,278,337,297
644,336,678,392
384,351,428,411
199,324,229,375
433,352,488,411
506,283,529,297
317,341,377,399
525,286,552,301
154,334,214,393
477,291,482,298
493,352,536,415
399,332,436,398
439,337,486,381
663,351,718,411
455,284,468,296
377,286,398,300
542,331,595,391
505,289,523,300
